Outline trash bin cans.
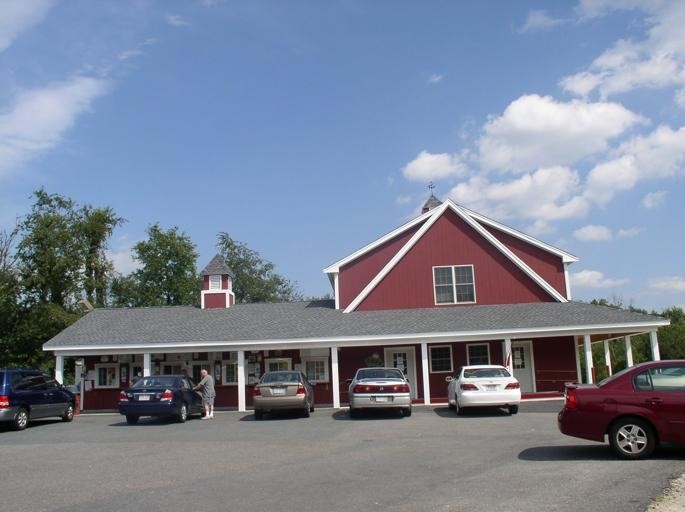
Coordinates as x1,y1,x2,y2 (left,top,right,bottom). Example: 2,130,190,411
66,385,81,417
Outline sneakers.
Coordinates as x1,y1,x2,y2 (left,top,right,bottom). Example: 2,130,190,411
201,415,214,419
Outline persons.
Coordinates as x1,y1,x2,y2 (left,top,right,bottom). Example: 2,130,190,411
190,369,216,421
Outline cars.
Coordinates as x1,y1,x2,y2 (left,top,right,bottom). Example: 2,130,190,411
252,370,319,419
118,374,205,424
555,358,685,458
445,364,521,415
636,367,685,389
345,365,414,418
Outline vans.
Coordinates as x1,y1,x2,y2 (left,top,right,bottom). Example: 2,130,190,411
0,367,77,430
